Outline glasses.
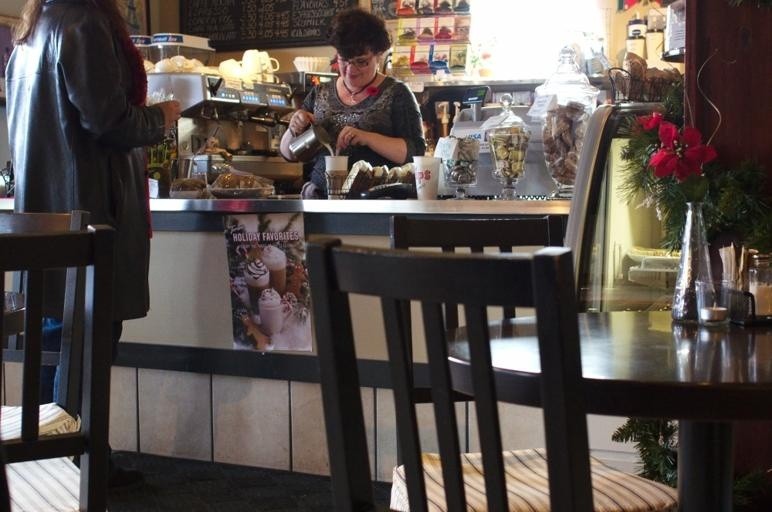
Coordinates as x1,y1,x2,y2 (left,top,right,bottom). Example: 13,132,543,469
337,54,374,66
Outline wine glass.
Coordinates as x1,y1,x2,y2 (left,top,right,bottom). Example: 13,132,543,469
442,139,482,200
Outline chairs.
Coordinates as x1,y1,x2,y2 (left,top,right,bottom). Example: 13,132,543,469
1,209,118,512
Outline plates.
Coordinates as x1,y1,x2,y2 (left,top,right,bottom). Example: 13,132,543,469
211,186,273,197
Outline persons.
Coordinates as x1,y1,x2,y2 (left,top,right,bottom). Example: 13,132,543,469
279,7,426,198
5,0,180,489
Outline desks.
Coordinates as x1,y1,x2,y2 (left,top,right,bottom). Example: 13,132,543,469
445,310,771,511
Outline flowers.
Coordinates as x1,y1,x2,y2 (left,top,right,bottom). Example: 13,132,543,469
617,48,771,322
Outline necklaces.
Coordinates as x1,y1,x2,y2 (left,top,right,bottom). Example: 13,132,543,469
342,74,380,103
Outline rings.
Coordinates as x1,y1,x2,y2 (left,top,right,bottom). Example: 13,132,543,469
345,134,348,139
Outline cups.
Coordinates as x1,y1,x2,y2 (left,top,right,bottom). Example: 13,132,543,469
244,257,293,334
694,278,730,327
412,155,441,200
324,155,350,199
237,49,280,82
645,29,663,59
289,120,331,162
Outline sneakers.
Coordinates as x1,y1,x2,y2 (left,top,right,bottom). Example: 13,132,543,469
108,461,143,489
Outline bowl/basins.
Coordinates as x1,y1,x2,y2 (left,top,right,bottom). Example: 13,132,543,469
294,56,330,71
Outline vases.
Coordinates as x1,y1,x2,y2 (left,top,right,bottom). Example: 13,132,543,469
671,201,716,324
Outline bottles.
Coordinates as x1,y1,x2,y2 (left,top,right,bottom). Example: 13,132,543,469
749,253,770,319
537,45,601,202
485,94,533,200
624,12,647,61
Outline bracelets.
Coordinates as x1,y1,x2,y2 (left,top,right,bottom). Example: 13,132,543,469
289,128,295,136
